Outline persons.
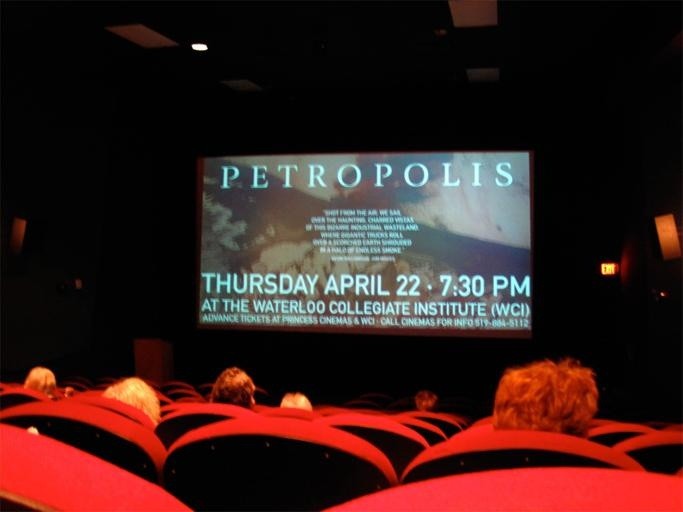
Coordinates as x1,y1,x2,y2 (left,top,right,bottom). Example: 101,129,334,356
280,391,313,413
18,365,58,402
102,375,164,430
408,389,438,411
204,365,259,411
488,355,602,440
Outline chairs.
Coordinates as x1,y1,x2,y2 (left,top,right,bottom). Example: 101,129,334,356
0,370,682,511
